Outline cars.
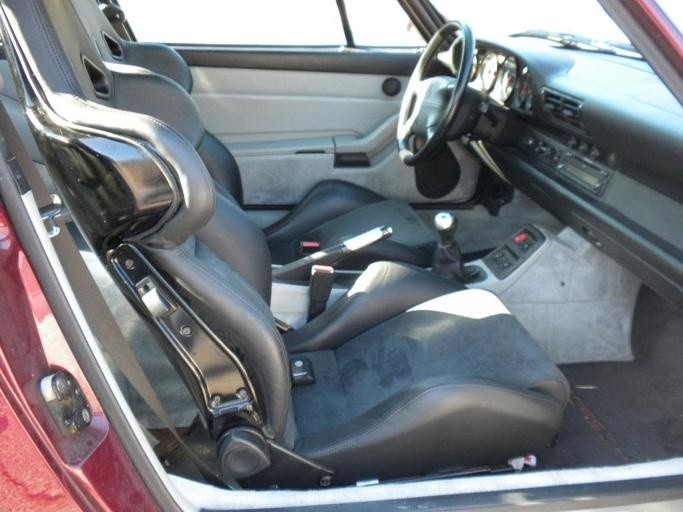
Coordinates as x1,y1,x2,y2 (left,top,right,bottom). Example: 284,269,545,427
0,0,680,512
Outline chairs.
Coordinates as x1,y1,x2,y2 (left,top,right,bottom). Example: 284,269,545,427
1,0,572,490
70,0,440,281
